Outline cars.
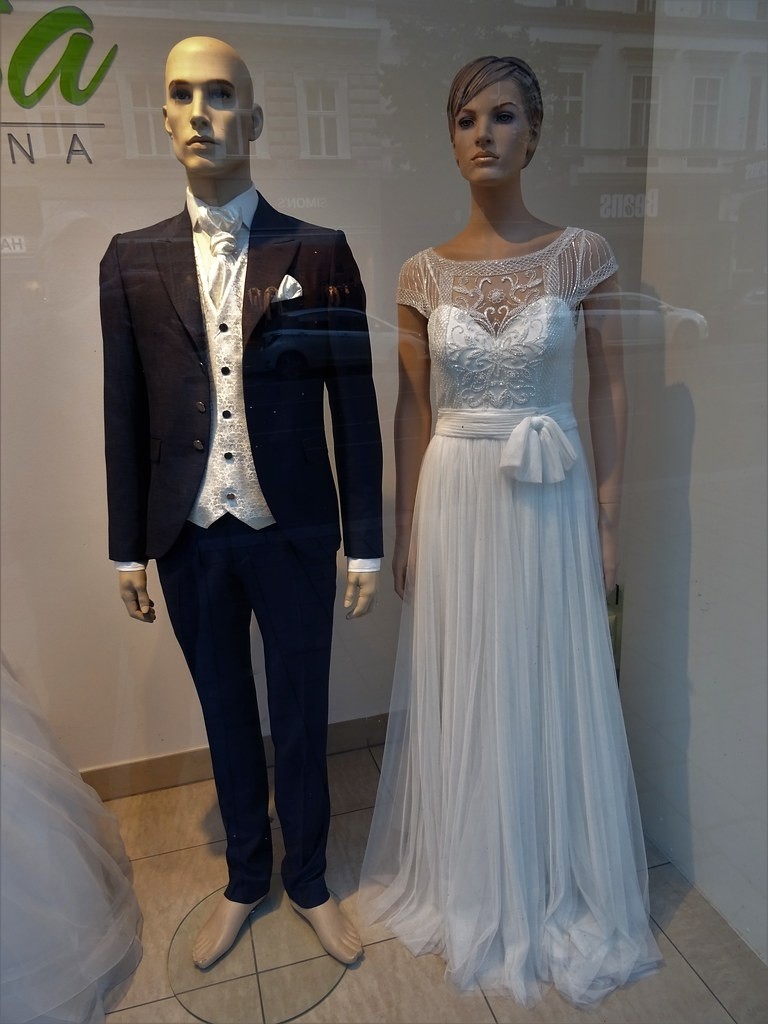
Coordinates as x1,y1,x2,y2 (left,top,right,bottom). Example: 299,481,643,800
256,302,430,386
581,292,712,352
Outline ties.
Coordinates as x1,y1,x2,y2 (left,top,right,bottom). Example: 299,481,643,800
195,203,243,308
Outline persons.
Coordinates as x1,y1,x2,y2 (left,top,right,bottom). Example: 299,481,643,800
99,36,385,968
356,54,662,1014
